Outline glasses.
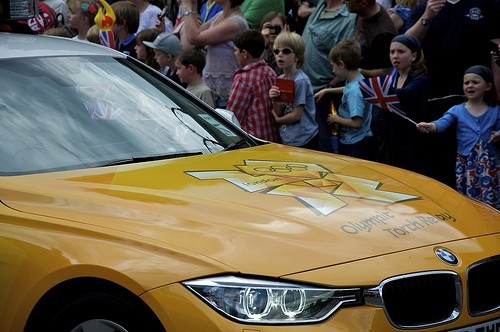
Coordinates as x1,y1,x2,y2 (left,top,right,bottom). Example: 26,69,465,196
274,48,295,54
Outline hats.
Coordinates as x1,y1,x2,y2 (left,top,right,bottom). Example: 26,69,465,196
142,32,182,56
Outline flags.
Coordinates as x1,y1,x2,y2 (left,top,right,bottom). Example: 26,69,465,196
93,99,124,120
359,75,406,117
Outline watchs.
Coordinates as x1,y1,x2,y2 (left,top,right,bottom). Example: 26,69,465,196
182,9,190,16
327,84,335,88
419,16,432,25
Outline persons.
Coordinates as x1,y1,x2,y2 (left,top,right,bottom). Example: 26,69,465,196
127,0,173,37
104,2,145,63
225,30,282,144
173,0,250,110
315,40,375,161
269,30,320,151
240,0,287,78
135,27,187,88
0,0,79,39
175,47,216,109
416,65,500,213
403,0,500,189
383,34,431,172
285,0,417,150
66,0,101,45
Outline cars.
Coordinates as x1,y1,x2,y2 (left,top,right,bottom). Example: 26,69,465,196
0,30,499,332
